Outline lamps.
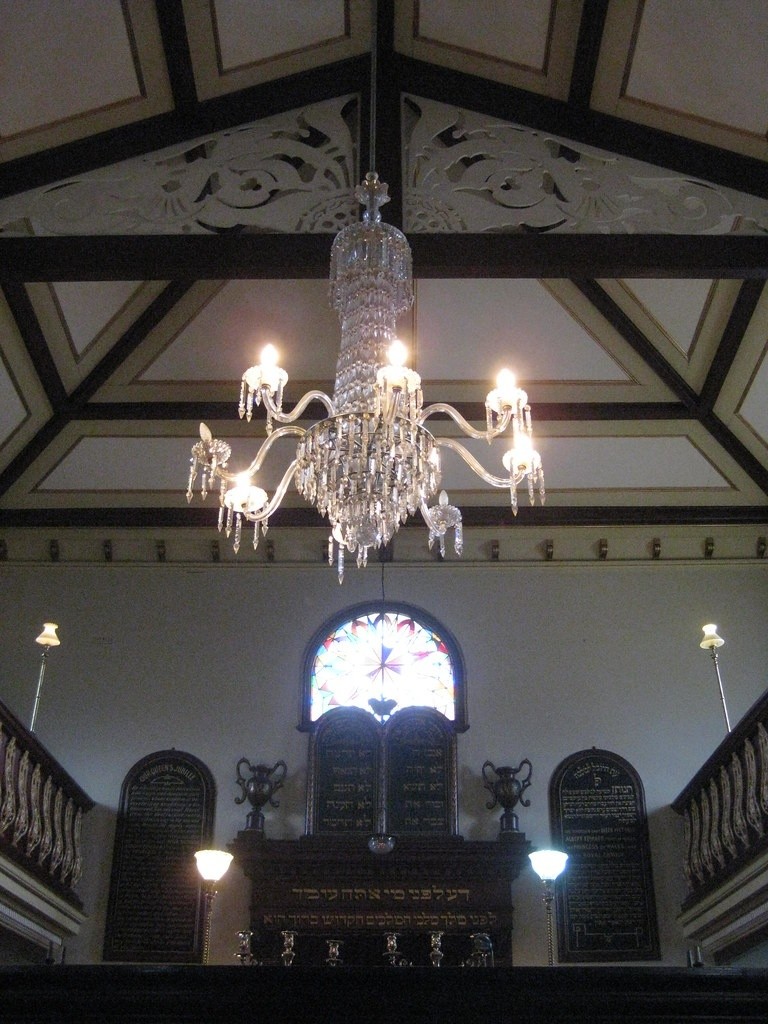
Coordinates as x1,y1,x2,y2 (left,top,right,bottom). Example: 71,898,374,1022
701,623,732,732
187,7,548,585
195,849,233,964
29,623,60,731
529,850,570,966
